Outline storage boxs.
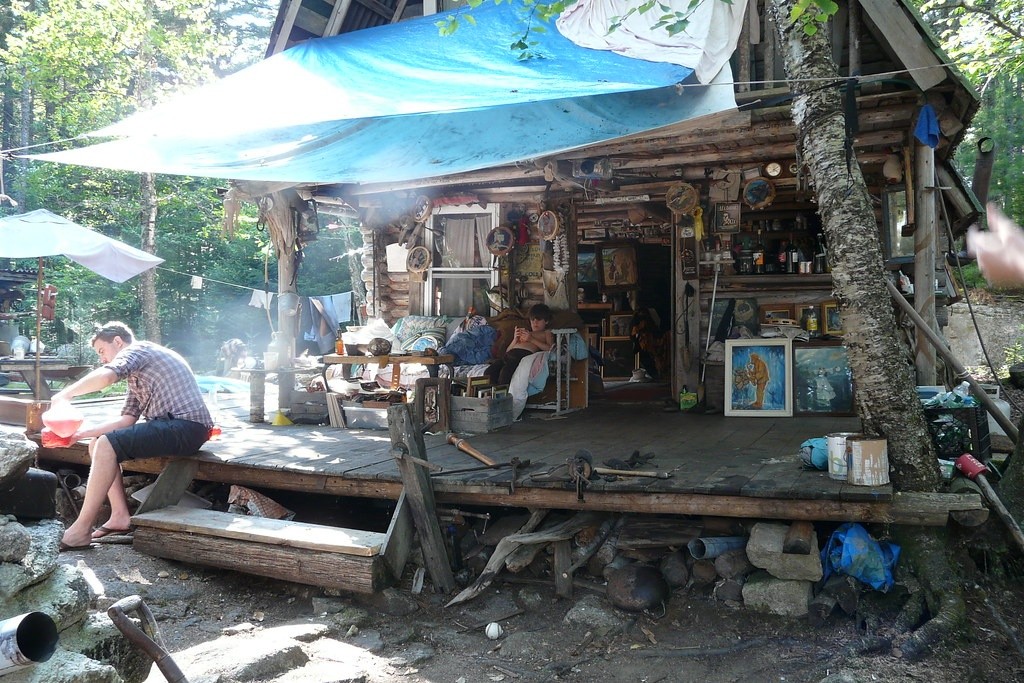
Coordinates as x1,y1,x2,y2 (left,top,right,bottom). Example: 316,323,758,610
290,391,388,429
923,402,992,463
451,393,513,433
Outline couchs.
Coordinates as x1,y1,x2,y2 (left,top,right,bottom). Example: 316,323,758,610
364,315,588,417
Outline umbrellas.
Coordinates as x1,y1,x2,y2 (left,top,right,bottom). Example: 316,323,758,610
0,208,166,401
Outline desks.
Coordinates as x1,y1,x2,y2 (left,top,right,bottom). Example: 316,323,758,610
0,359,68,400
323,354,453,431
231,367,310,423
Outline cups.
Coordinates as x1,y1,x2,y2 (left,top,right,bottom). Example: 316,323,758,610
955,452,989,479
901,284,914,294
263,352,279,369
798,261,812,277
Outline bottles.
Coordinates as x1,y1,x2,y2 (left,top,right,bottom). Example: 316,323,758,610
923,380,977,407
602,292,607,303
681,385,687,394
806,306,818,338
334,329,344,354
445,526,462,570
701,211,827,277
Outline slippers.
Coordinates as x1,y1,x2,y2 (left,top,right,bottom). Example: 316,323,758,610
59,541,96,552
91,526,137,541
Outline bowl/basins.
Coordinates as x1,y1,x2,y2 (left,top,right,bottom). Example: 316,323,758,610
41,410,84,438
632,368,646,379
345,343,368,356
346,325,366,332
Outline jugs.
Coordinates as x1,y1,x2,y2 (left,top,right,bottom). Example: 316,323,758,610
268,330,289,367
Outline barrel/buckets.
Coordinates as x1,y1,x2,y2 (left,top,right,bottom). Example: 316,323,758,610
845,435,889,486
823,432,864,481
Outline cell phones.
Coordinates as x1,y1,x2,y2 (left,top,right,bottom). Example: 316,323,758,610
519,328,525,339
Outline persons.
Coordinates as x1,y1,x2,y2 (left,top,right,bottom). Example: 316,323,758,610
49,320,214,552
964,202,1024,290
483,303,554,393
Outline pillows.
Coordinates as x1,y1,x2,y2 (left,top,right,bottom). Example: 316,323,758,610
401,315,488,354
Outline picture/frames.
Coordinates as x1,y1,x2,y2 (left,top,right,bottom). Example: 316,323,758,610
724,302,856,418
415,376,509,434
577,244,643,381
